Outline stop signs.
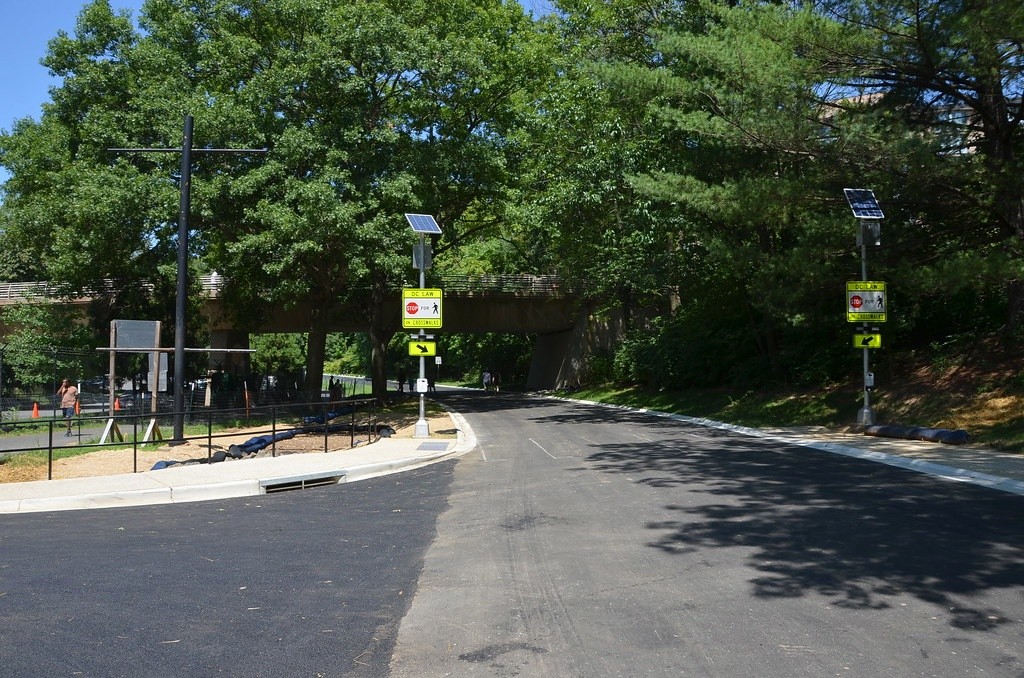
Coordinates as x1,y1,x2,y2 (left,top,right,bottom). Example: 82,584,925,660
850,295,863,309
406,302,419,315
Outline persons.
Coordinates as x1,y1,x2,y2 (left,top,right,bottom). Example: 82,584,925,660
492,369,501,396
334,379,344,393
329,375,335,399
408,375,415,393
57,378,79,437
397,367,406,391
427,371,437,394
482,367,491,395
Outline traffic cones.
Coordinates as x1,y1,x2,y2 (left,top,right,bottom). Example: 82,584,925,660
115,397,121,410
31,402,41,419
75,402,81,415
